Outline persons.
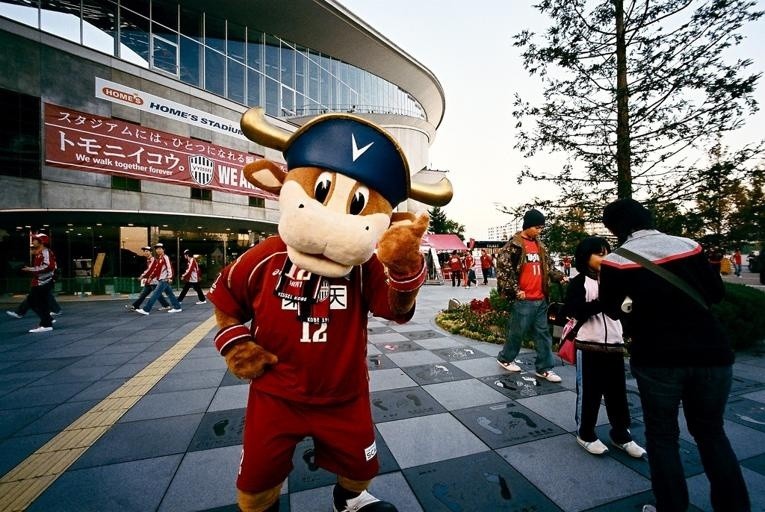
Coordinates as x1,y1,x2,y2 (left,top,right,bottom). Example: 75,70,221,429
21,233,57,332
136,243,182,315
497,209,569,382
565,238,647,459
733,250,742,278
600,199,751,512
177,249,207,305
748,252,755,269
448,249,497,288
563,254,573,277
125,247,171,312
6,242,63,319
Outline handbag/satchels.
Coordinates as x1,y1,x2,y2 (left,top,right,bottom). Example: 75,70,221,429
557,319,577,367
548,299,575,326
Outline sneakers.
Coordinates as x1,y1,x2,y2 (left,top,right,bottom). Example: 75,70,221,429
123,304,137,312
534,369,564,383
28,324,55,333
195,300,207,305
135,307,151,315
574,433,608,455
611,438,650,460
451,281,488,288
6,309,23,319
47,308,63,325
157,305,172,311
496,359,522,373
167,307,184,314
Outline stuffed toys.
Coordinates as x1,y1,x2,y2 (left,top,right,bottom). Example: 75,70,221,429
207,105,453,512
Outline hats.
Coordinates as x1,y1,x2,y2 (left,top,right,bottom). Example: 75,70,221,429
141,246,153,252
154,242,165,248
523,210,546,231
181,248,193,255
29,232,51,249
